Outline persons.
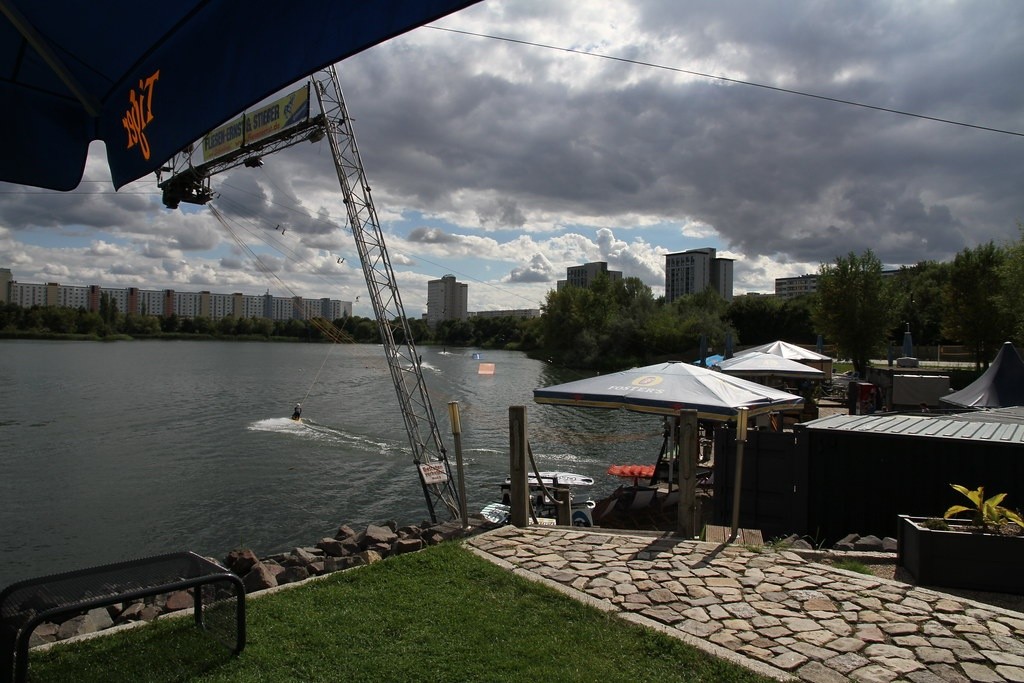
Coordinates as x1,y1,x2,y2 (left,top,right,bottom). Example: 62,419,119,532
920,402,931,413
293,403,302,419
864,393,888,412
798,378,817,409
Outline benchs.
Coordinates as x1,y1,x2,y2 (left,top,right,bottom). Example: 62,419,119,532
0,551,246,682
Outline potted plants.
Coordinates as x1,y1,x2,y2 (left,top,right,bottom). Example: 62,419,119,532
896,484,1024,596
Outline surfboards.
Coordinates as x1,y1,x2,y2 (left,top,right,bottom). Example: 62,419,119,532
504,471,595,486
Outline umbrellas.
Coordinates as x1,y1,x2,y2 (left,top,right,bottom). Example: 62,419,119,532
725,333,733,360
700,336,707,367
903,332,912,356
533,361,804,491
817,335,823,353
711,340,833,389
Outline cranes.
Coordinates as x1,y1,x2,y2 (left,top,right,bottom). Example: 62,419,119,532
156,63,463,527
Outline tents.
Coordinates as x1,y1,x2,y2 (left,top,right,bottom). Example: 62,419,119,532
940,342,1024,412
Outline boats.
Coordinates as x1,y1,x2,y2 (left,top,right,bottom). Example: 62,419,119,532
505,469,594,487
